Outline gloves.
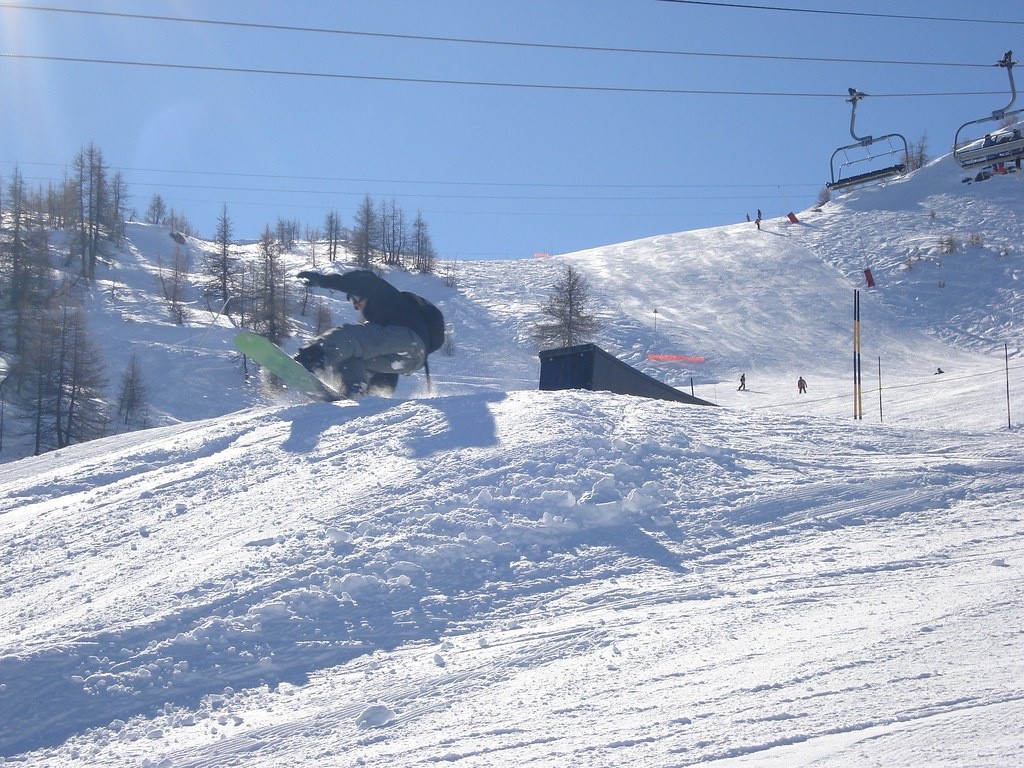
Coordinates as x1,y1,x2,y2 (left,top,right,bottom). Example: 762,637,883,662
297,271,321,286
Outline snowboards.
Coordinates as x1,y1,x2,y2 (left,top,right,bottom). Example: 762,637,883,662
233,330,349,403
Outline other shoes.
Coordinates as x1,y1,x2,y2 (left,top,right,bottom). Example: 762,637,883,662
338,382,364,399
295,346,326,376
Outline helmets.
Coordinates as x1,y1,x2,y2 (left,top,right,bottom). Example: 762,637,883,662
346,292,361,304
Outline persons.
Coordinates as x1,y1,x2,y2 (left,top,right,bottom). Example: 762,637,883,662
934,367,944,375
798,377,807,394
755,219,760,230
265,268,445,400
738,373,746,390
757,208,762,220
1008,128,1024,169
982,133,1005,172
745,213,750,222
